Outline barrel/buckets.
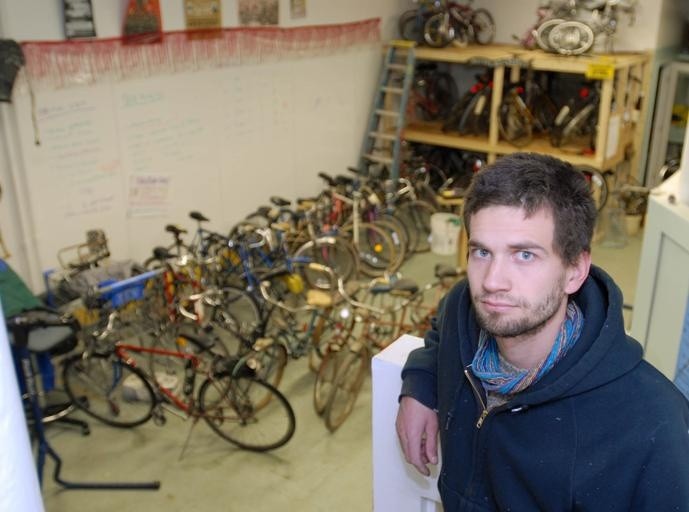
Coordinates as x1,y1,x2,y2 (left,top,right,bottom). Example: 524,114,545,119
430,212,461,255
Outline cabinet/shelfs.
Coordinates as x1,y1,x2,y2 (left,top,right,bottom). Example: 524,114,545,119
381,40,689,212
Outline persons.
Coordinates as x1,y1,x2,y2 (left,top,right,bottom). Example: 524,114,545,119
394,151,688,511
